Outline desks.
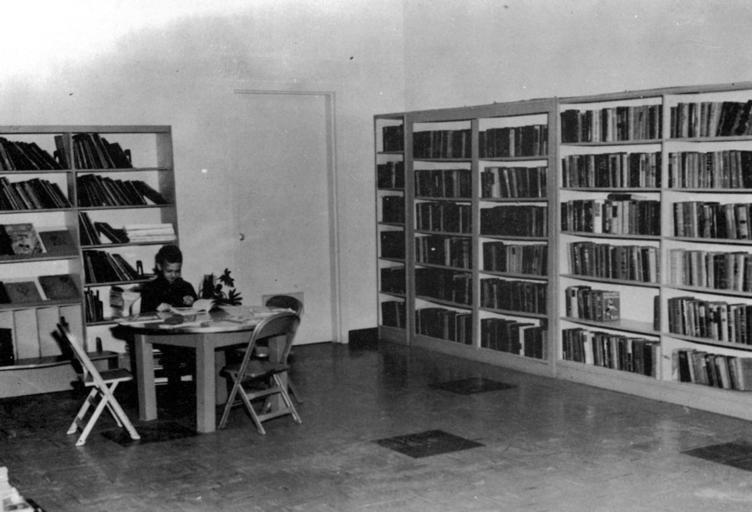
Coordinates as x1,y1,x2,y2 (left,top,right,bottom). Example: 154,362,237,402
116,306,285,434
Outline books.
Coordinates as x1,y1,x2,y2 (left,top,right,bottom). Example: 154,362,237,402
478,124,548,359
560,104,662,283
668,100,752,394
171,297,214,316
562,286,661,380
412,129,472,345
378,124,406,329
1,132,168,367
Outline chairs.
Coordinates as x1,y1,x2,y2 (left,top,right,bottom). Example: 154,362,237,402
57,323,141,447
219,313,301,436
242,294,303,408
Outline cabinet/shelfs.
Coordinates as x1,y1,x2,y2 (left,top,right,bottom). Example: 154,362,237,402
559,82,750,424
372,97,557,381
0,123,192,399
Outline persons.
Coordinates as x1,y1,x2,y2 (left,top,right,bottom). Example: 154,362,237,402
143,244,199,405
